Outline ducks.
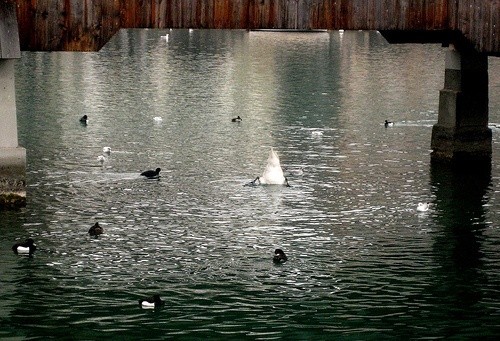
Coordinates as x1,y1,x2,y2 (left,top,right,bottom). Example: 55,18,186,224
80,115,88,122
97,155,105,161
88,222,104,233
139,168,162,178
231,116,243,122
138,294,162,307
103,146,112,153
272,249,288,262
417,202,428,211
384,120,395,124
12,238,37,250
